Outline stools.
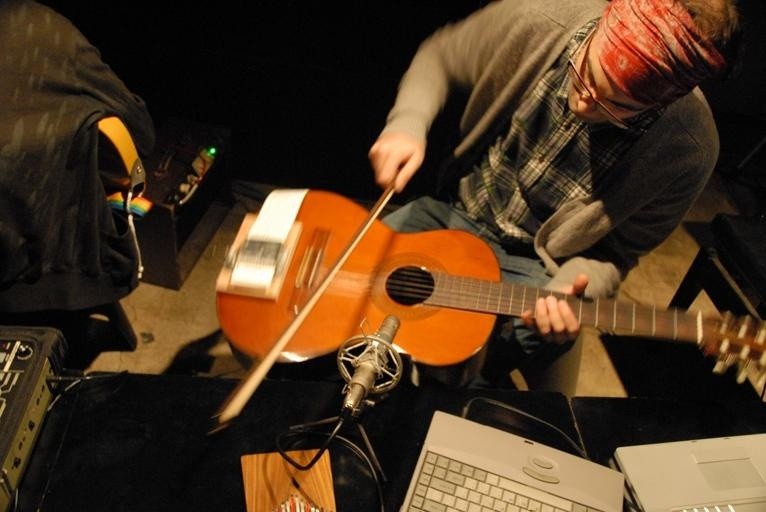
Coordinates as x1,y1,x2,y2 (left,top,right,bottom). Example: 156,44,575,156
669,212,766,320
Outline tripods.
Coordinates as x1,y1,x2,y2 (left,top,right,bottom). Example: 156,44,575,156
290,399,398,486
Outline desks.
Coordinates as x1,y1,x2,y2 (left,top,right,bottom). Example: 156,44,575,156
11,369,766,510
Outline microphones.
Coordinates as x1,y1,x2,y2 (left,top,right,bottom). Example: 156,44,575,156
339,313,401,419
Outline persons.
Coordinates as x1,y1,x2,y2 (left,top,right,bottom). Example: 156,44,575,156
275,0,747,389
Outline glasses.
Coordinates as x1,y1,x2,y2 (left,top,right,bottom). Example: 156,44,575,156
565,21,658,133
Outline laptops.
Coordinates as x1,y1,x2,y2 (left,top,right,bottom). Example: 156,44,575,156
613,433,766,512
396,410,625,512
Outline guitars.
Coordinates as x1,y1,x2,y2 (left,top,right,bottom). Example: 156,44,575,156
218,189,760,366
